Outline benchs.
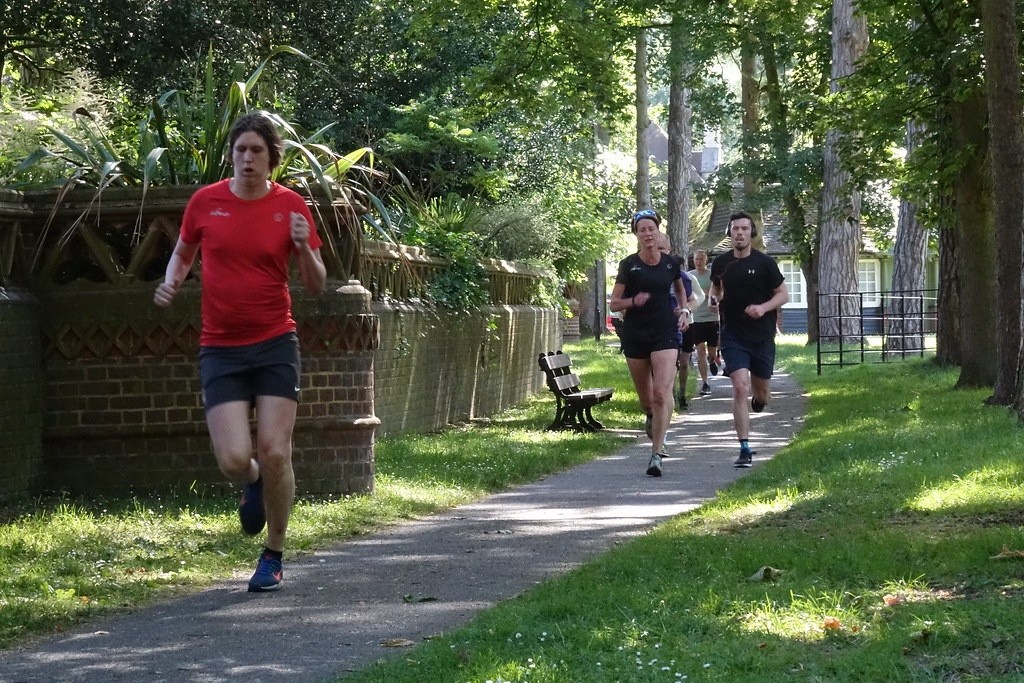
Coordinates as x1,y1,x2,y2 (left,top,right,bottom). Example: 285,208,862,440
539,351,614,432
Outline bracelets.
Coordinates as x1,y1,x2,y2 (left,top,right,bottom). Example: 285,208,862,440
631,296,636,306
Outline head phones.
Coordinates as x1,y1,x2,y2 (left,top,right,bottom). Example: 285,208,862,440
725,222,757,238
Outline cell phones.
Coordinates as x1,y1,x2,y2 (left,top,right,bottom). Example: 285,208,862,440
711,296,716,305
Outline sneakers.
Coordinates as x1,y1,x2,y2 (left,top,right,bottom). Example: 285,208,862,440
646,453,663,477
645,414,653,438
678,391,690,411
247,553,284,591
751,396,767,412
699,383,713,394
661,442,669,457
733,447,753,467
238,475,266,536
707,356,718,376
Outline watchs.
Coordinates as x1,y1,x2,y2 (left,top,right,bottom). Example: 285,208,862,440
680,308,691,317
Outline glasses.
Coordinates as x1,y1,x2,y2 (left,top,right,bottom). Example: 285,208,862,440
631,209,660,234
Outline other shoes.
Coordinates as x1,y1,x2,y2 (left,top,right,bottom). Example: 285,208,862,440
715,354,722,367
689,360,694,366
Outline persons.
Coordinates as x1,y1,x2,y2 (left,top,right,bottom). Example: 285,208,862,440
154,112,327,592
707,212,789,468
610,210,691,478
658,233,730,411
